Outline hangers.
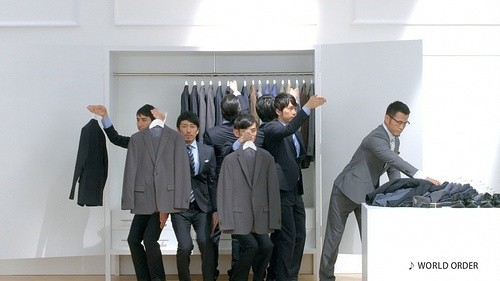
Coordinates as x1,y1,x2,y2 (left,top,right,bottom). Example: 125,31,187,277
148,118,164,130
91,111,98,120
184,74,314,86
242,141,257,151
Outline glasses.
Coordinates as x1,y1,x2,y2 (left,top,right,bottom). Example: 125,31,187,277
387,114,410,126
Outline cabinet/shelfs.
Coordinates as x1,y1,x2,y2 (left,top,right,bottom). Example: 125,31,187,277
104,207,322,281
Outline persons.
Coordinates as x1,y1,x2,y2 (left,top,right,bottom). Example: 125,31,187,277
263,93,327,281
255,96,277,148
151,109,218,281
204,80,249,281
221,114,275,281
87,104,170,281
319,101,441,281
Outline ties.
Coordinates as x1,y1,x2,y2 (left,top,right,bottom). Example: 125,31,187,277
384,139,395,169
292,133,300,158
186,146,196,203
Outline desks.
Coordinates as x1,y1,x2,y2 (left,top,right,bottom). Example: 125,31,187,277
361,203,500,281
